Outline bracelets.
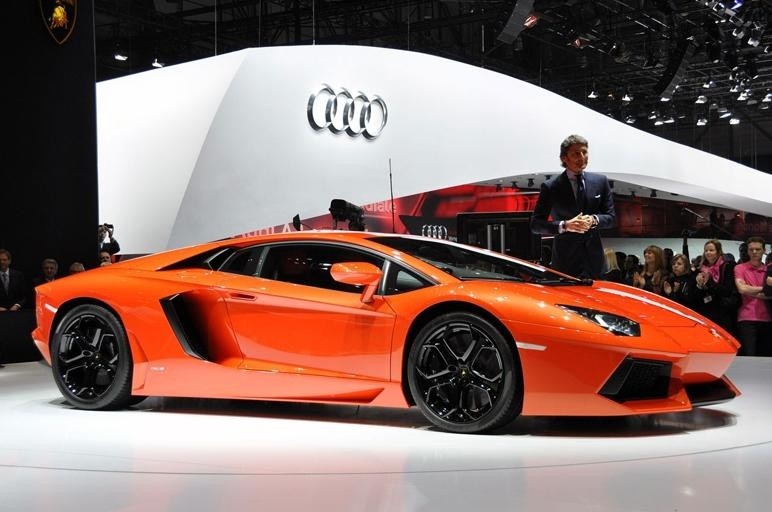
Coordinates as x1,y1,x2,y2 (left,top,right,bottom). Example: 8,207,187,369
561,220,567,233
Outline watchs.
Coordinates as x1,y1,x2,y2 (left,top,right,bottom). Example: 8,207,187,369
590,215,599,230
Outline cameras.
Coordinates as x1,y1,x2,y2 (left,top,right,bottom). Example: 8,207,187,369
104,223,113,228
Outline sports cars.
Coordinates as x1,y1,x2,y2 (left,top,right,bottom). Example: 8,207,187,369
24,228,748,439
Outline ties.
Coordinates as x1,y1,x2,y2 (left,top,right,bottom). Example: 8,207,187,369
574,174,587,214
2,272,9,295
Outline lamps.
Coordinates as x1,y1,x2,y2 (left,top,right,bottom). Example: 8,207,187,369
523,0,771,131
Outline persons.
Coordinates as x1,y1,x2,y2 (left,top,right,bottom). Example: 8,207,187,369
602,234,772,358
30,257,62,291
97,249,113,267
97,222,120,259
529,132,618,280
67,261,86,275
534,244,553,268
0,249,29,313
